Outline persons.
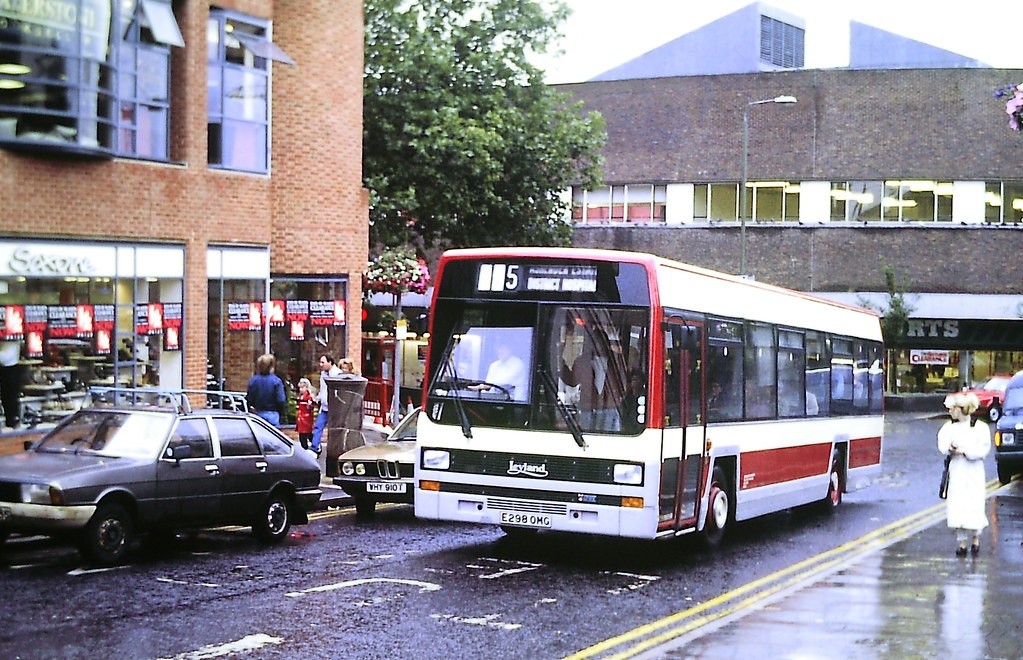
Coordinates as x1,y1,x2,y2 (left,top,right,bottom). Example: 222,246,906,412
338,358,353,373
557,329,627,431
246,355,285,428
807,392,818,415
911,364,929,393
298,378,315,449
709,381,727,408
937,391,991,555
309,353,343,458
854,381,863,399
485,342,525,400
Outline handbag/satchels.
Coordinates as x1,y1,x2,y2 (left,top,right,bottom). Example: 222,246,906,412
939,470,949,499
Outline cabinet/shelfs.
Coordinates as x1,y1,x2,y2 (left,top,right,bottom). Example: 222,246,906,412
16,339,152,418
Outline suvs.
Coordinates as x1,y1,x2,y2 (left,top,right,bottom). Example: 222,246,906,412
995,371,1023,485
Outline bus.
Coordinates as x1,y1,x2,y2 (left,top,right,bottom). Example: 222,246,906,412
411,246,884,545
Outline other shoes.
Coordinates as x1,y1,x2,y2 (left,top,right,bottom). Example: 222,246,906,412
317,443,322,453
955,547,967,555
971,544,979,553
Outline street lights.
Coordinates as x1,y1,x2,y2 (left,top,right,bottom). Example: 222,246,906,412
740,95,797,279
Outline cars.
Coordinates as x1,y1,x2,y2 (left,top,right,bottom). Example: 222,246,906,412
0,390,323,566
333,407,415,515
962,374,1012,422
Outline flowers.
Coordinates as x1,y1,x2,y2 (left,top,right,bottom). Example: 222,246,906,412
362,244,431,299
992,82,1023,134
